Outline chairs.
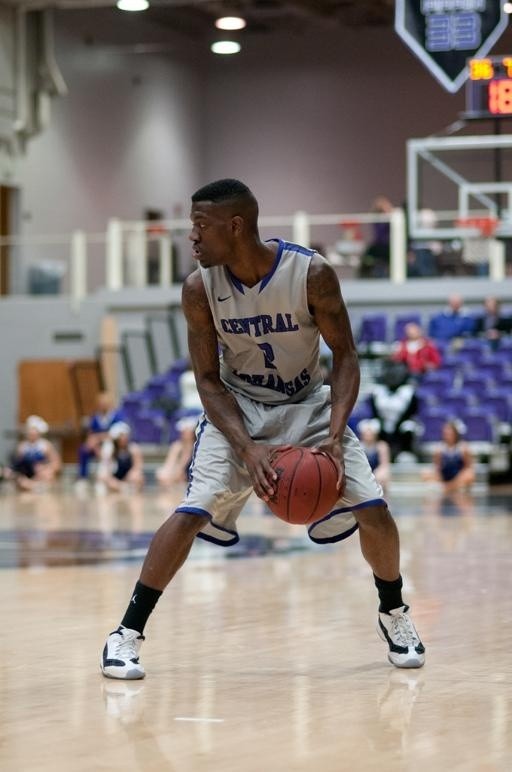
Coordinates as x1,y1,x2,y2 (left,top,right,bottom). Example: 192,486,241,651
117,312,512,451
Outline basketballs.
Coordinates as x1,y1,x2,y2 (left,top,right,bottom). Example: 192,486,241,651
261,445,340,526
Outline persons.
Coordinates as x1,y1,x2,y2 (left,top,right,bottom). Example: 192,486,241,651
470,294,509,346
427,294,473,344
157,412,200,481
404,207,445,276
103,179,426,679
360,195,408,279
72,392,128,490
352,418,392,503
363,352,420,465
100,421,145,492
426,416,477,503
0,412,60,493
392,322,437,386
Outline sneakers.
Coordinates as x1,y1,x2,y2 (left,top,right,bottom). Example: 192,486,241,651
374,603,425,669
100,628,147,679
